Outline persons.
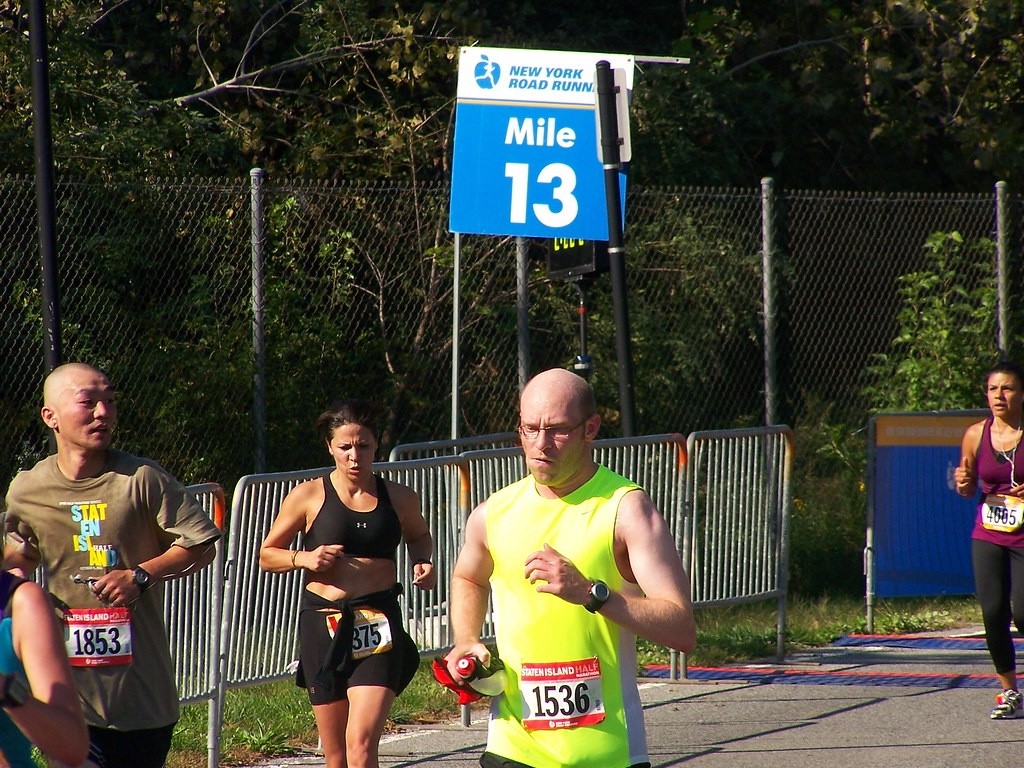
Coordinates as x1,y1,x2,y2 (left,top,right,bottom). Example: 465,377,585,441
443,368,697,768
955,362,1024,720
0,569,91,768
0,363,222,768
260,401,435,768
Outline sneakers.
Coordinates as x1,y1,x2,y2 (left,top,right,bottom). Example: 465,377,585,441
990,688,1024,719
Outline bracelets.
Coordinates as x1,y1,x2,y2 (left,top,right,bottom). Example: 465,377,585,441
412,559,432,572
291,550,299,569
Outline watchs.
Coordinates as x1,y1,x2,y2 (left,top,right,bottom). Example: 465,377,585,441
583,579,610,613
0,677,26,707
130,566,150,592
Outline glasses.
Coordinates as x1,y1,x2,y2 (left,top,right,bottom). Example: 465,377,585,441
516,416,591,440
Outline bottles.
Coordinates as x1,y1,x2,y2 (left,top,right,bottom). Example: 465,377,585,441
456,655,506,698
